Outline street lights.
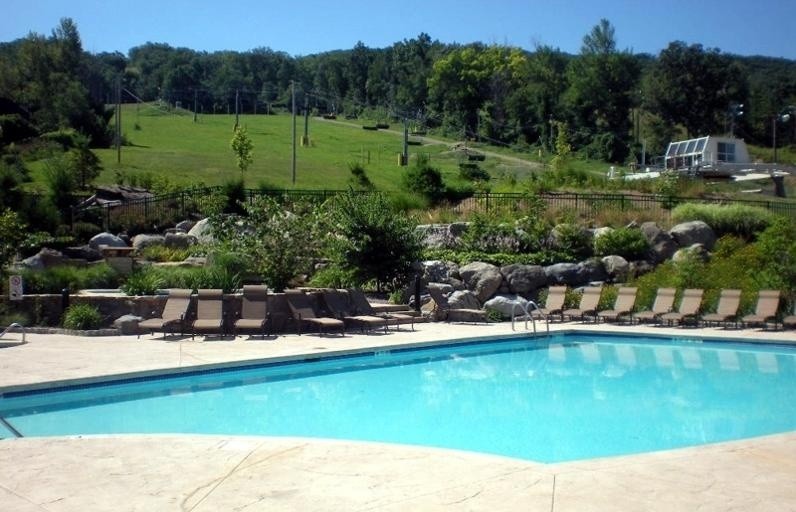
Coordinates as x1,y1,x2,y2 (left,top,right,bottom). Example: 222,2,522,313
771,111,791,165
727,99,746,137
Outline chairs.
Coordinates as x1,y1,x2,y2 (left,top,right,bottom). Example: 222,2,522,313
521,282,796,332
136,284,415,342
